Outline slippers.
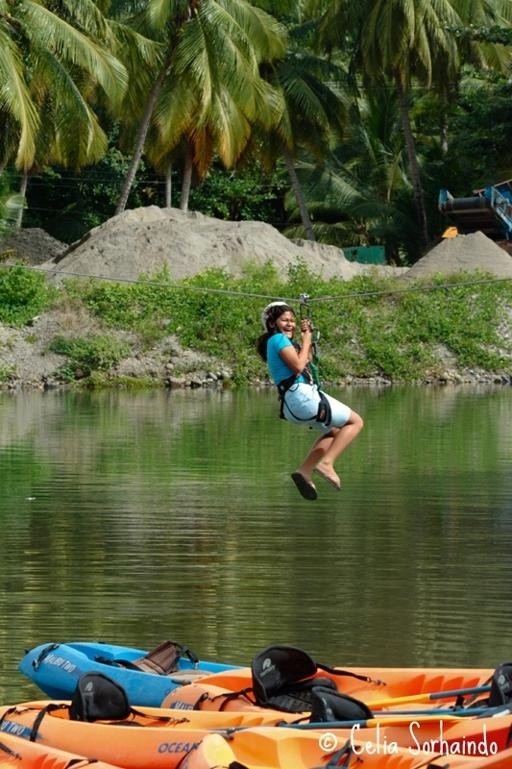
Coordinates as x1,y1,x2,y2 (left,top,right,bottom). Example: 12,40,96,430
313,468,341,492
290,472,317,501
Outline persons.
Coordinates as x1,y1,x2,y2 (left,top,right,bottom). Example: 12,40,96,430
260,299,364,501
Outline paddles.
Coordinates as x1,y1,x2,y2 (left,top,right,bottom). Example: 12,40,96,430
362,685,492,708
275,715,469,729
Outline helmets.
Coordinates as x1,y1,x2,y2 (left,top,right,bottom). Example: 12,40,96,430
261,301,289,334
